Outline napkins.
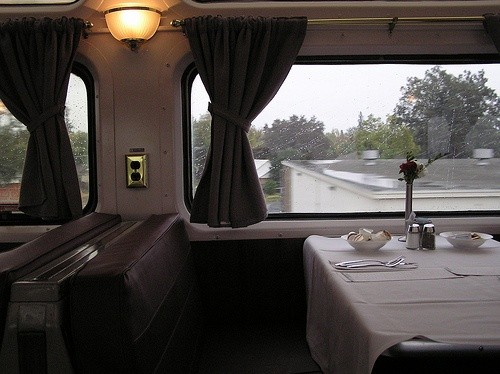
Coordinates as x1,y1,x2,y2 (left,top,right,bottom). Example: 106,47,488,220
345,268,460,281
443,266,500,277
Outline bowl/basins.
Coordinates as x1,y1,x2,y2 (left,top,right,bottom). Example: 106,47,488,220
341,233,393,251
439,231,493,249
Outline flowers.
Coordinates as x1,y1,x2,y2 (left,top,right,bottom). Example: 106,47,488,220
397,151,448,183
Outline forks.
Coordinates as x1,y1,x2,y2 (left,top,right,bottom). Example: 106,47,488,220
444,266,500,276
335,255,406,268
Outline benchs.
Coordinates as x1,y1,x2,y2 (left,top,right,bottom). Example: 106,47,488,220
0,214,319,374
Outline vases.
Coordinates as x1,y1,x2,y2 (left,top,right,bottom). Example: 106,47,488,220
398,184,413,243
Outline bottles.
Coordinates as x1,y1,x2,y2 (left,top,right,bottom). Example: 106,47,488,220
406,224,436,251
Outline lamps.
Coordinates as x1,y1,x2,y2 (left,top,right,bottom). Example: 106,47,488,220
103,7,161,51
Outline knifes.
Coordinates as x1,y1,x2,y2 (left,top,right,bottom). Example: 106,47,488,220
335,259,417,266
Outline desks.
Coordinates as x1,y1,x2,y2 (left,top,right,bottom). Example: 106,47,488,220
304,233,500,374
